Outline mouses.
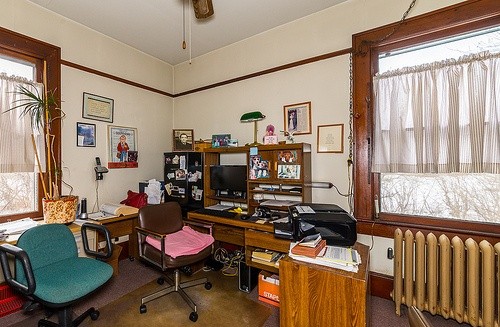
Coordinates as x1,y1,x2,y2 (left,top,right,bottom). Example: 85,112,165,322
241,215,249,220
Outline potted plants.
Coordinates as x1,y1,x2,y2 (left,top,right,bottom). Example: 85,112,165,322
3,84,80,226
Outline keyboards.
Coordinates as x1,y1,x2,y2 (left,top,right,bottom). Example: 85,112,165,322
195,208,238,218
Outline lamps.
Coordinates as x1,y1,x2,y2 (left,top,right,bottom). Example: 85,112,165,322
243,112,264,146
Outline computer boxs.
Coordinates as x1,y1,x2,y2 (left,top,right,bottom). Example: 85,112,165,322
239,257,262,294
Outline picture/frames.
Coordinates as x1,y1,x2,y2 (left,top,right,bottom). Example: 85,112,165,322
82,92,114,123
284,103,310,135
108,124,138,163
172,130,194,152
317,124,343,154
76,122,96,147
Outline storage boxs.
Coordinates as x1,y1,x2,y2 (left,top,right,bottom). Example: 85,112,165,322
257,270,280,308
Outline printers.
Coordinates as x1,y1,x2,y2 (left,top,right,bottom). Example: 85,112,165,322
274,203,357,246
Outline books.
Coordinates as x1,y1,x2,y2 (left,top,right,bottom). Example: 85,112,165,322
251,250,285,266
299,233,322,247
88,203,139,221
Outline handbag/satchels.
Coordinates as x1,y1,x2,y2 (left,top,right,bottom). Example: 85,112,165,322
120,190,148,209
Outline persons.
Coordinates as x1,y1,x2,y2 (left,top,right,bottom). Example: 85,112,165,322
174,133,192,150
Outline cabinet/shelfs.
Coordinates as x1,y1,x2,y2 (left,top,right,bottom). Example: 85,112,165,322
164,142,368,327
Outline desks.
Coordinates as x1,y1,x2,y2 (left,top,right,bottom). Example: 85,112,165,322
78,210,138,260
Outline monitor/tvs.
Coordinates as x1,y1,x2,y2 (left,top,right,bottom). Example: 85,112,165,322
210,164,247,199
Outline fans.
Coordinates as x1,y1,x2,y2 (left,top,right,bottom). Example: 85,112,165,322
193,0,214,18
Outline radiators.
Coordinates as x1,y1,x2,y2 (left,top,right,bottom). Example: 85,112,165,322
394,228,500,327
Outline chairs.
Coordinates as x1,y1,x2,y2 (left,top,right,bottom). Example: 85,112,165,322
135,201,214,322
0,222,114,327
